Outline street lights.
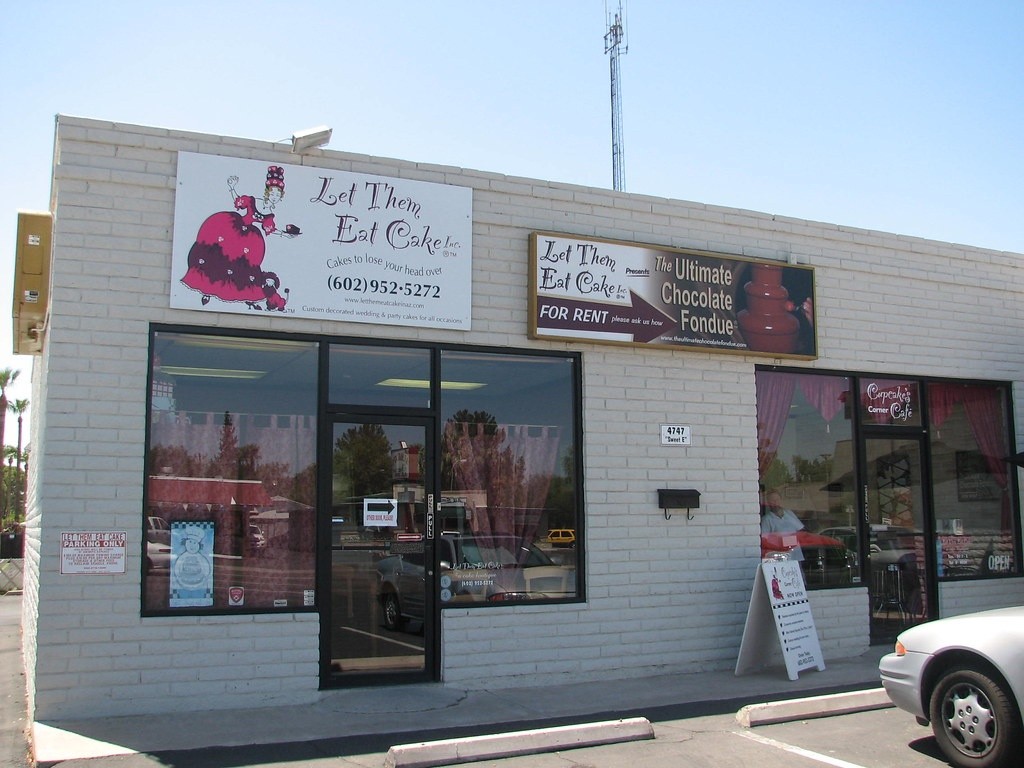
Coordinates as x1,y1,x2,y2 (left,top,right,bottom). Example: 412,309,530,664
450,459,467,490
820,454,831,482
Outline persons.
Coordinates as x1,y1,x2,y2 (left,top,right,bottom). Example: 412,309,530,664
760,490,805,588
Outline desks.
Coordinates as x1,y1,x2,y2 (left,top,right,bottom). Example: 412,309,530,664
327,568,378,639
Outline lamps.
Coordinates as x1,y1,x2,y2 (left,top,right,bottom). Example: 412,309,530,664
273,126,333,152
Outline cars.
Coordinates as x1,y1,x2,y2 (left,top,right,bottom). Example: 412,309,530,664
879,606,1024,768
249,525,266,550
375,530,574,630
539,529,577,548
147,540,178,570
146,516,170,535
760,501,856,589
790,525,924,598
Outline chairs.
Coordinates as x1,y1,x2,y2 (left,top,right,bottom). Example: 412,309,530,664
442,565,571,600
